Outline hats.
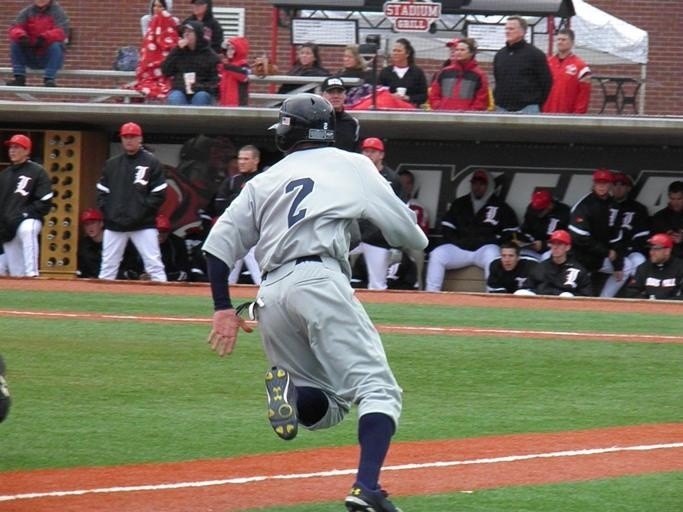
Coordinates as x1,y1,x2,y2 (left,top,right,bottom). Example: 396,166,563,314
446,38,462,47
120,122,142,136
531,190,551,210
612,172,632,184
592,169,614,182
646,234,673,249
360,137,384,152
4,134,32,150
155,214,172,230
469,171,488,183
80,207,103,223
546,230,573,245
322,76,345,92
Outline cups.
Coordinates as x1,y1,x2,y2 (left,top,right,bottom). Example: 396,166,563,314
182,71,198,94
396,86,408,98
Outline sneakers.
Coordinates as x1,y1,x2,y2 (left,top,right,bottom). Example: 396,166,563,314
7,75,27,86
44,77,57,87
344,480,402,512
263,365,299,441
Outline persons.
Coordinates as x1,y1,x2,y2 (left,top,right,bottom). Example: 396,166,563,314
273,16,591,112
201,92,431,512
6,0,71,86
137,0,249,107
343,135,683,300
79,119,271,287
0,134,54,277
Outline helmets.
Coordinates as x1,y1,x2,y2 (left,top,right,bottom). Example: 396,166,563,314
265,92,338,153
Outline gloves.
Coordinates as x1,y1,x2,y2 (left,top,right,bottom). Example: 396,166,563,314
21,37,29,53
35,36,45,49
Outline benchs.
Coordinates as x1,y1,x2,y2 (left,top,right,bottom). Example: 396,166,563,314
186,214,683,302
